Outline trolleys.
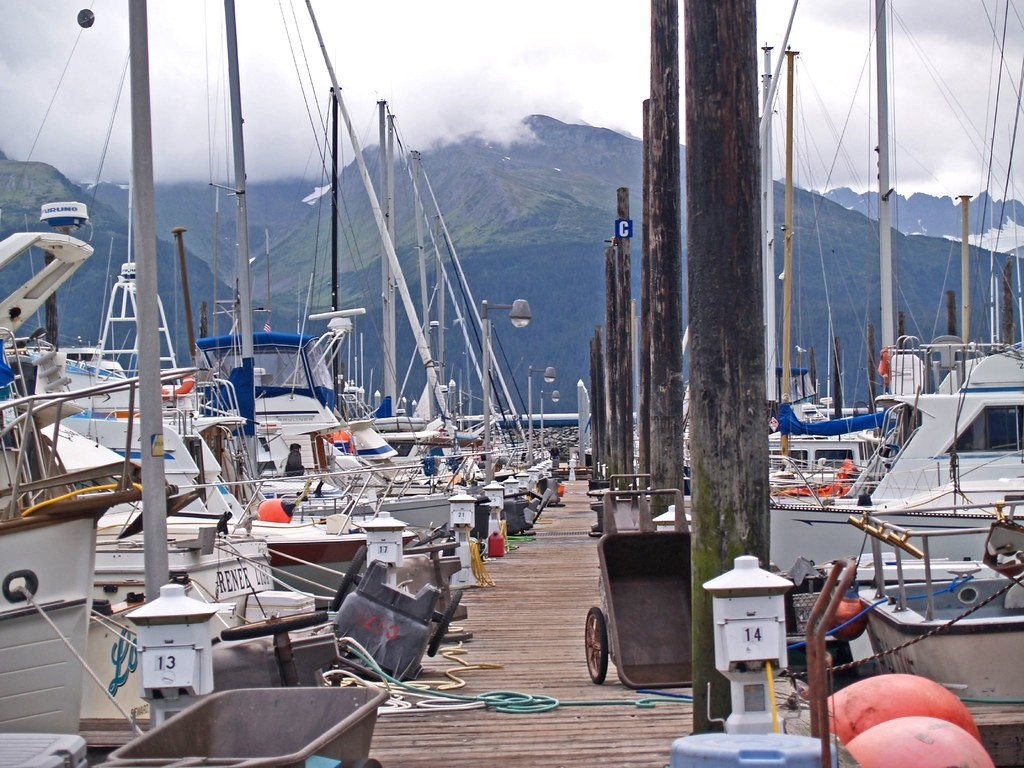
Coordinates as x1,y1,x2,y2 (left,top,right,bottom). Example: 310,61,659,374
330,541,464,683
584,473,693,690
93,686,389,768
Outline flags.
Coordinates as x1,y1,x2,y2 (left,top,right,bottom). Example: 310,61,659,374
264,322,271,332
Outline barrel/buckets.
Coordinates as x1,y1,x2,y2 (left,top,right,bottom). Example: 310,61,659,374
557,484,565,497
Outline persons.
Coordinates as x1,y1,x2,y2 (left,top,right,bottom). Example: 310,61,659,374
280,443,307,477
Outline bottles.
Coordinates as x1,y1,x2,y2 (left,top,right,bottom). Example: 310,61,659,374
488,531,504,557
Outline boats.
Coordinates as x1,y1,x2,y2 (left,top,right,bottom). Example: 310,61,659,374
847,497,1024,708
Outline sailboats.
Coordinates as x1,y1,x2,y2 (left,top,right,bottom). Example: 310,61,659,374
757,0,1024,589
0,0,563,768
